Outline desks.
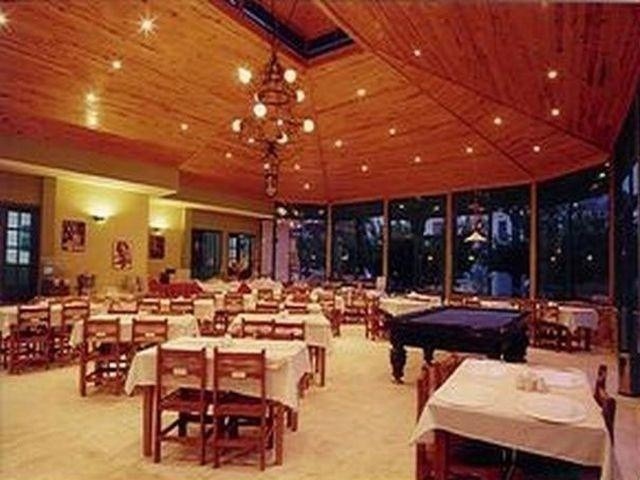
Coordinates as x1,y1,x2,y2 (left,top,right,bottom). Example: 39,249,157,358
389,305,533,384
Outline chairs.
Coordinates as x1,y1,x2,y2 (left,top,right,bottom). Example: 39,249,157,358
414,354,619,480
363,276,616,354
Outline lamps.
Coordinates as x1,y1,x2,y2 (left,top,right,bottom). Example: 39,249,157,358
232,0,318,199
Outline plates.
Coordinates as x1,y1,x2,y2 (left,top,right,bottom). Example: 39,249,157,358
532,364,586,388
516,392,587,424
